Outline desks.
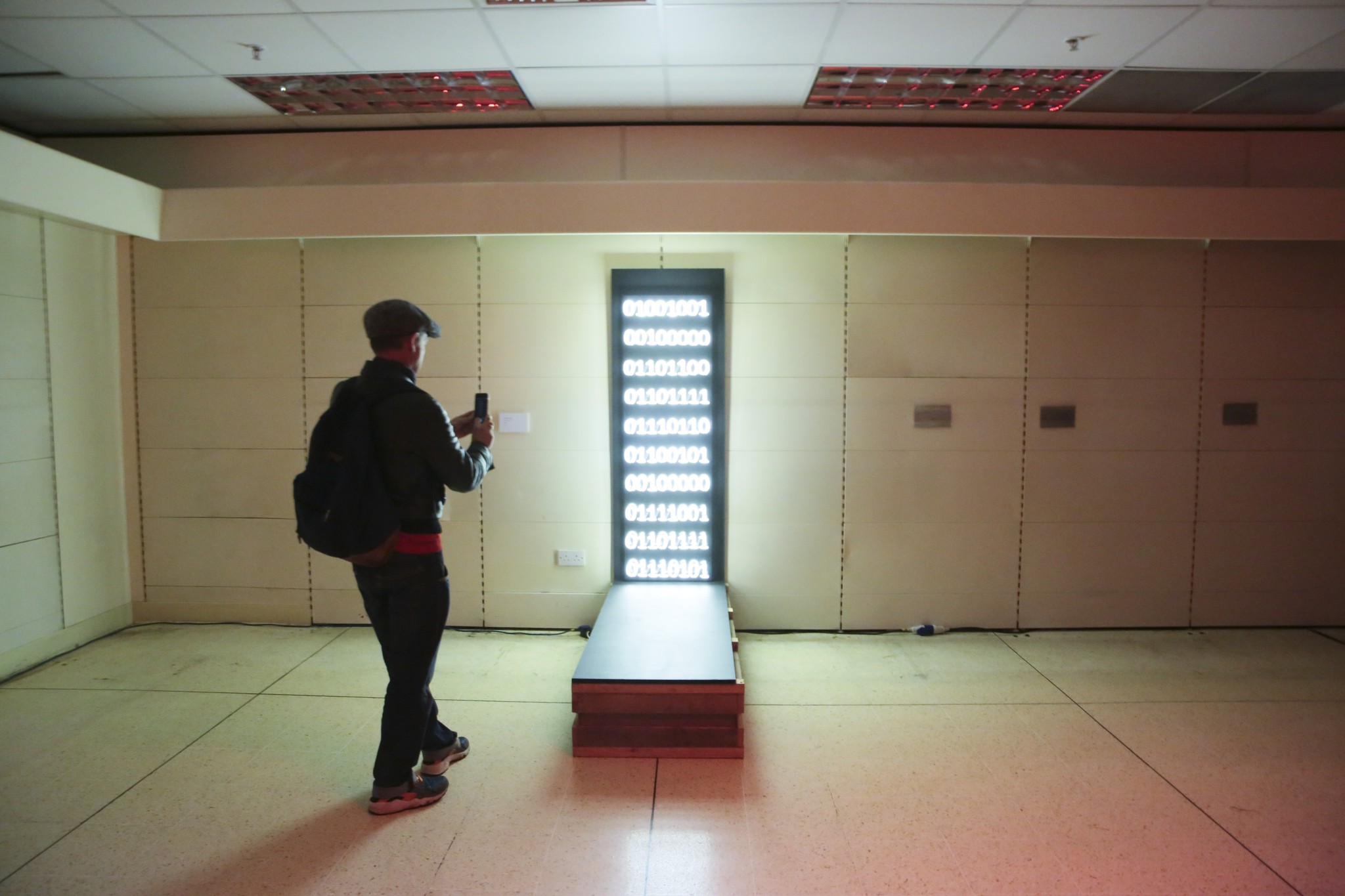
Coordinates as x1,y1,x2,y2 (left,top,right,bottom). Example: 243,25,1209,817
570,579,748,760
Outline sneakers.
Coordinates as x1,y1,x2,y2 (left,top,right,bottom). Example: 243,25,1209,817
369,774,451,815
420,736,470,775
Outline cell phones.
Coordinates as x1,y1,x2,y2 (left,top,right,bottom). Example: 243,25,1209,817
475,393,489,422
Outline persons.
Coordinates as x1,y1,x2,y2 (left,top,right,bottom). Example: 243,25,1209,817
297,300,503,812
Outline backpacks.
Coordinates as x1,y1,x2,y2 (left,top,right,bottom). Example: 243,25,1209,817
292,377,431,559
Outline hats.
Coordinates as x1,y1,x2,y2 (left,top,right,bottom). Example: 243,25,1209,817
364,299,441,339
